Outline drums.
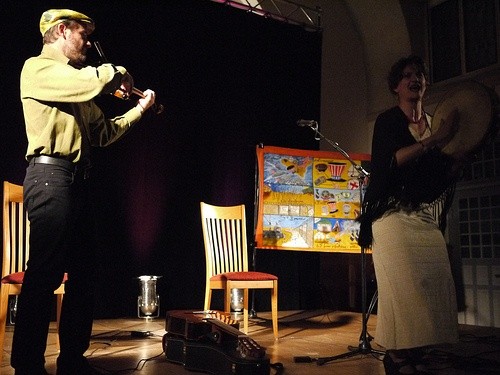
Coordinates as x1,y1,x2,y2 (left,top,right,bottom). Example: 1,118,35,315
431,83,499,157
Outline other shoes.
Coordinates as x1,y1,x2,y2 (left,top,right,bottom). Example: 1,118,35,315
15,368,49,375
56,363,100,375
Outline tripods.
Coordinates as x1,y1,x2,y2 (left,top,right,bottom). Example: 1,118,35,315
311,128,387,363
239,243,272,324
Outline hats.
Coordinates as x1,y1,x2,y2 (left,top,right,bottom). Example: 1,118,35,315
40,9,95,37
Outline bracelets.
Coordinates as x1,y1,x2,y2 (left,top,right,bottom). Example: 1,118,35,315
419,141,430,155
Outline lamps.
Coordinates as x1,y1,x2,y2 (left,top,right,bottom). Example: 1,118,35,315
230,288,244,315
138,275,163,322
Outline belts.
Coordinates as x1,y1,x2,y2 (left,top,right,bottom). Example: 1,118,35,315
30,156,89,181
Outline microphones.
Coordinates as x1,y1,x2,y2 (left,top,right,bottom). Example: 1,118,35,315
296,120,316,127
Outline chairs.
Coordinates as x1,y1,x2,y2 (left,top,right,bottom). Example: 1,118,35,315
0,182,68,366
201,200,280,341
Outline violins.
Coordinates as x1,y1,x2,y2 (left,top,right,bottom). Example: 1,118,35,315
77,60,164,115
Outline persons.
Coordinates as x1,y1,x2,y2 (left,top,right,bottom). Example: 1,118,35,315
10,9,155,375
363,58,465,375
